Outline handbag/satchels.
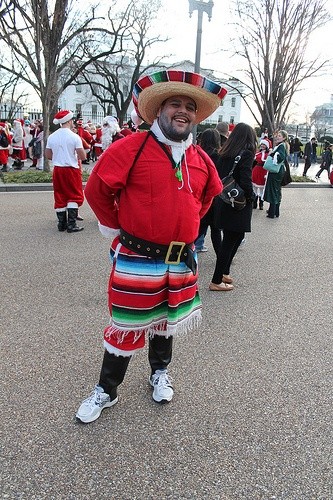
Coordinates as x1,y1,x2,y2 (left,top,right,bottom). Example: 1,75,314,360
218,151,248,210
263,155,283,174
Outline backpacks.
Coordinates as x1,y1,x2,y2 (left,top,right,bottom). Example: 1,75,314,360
0,130,8,147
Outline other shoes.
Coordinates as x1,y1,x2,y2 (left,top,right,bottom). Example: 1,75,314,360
209,282,234,290
196,247,208,252
77,216,84,220
259,206,263,210
223,276,233,283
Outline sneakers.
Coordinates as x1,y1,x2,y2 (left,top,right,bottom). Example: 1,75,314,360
149,369,174,403
76,385,118,423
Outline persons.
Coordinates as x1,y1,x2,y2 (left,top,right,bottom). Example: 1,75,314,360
253,127,290,218
74,115,136,165
44,110,87,232
310,142,318,163
74,71,227,425
195,121,235,252
302,138,316,176
287,138,303,168
208,123,253,290
0,118,44,171
316,140,333,186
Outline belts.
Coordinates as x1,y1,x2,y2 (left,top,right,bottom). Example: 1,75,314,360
119,227,189,265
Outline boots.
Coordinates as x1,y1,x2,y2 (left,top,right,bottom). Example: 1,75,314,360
66,202,84,233
56,207,67,231
2,160,22,172
82,160,89,164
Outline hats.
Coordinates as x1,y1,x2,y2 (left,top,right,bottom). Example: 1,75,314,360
132,71,228,124
53,110,72,125
261,138,272,149
103,116,118,128
